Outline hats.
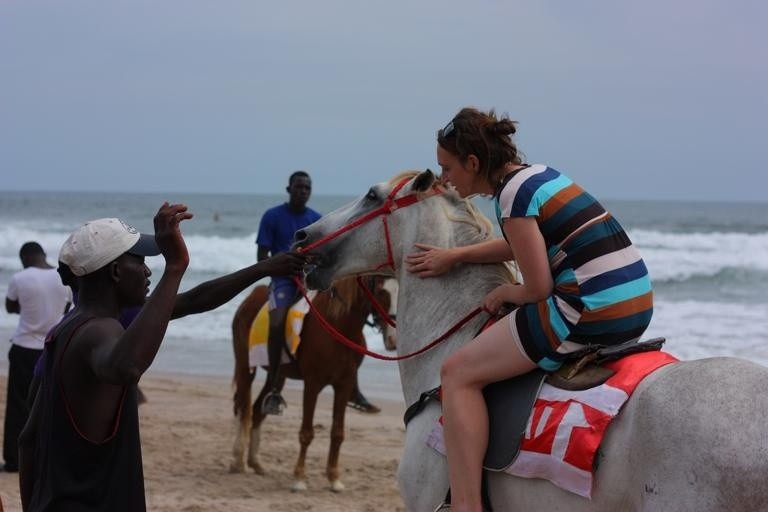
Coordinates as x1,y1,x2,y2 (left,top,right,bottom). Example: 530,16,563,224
57,218,162,285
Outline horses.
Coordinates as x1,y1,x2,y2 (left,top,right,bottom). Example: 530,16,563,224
230,272,401,495
292,167,768,512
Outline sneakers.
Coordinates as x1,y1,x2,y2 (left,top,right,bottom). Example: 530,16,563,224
346,392,380,413
264,394,282,415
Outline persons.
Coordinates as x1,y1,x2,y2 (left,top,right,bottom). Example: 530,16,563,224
403,107,653,512
255,171,382,413
19,201,306,512
1,242,73,473
